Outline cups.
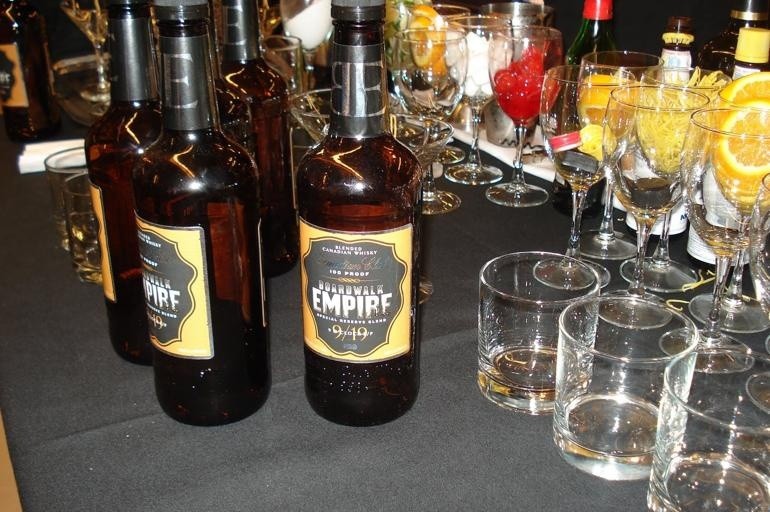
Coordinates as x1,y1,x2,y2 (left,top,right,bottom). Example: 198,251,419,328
645,348,769,512
60,174,104,284
477,250,601,416
289,89,333,143
44,146,90,252
257,34,304,131
551,297,700,481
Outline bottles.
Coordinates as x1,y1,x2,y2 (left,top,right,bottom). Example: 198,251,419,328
623,31,695,242
291,0,423,428
133,0,273,428
204,14,256,162
85,0,164,366
549,0,623,212
695,0,770,89
207,0,297,279
0,0,57,144
684,27,770,274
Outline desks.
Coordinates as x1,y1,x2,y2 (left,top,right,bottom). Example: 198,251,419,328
1,132,768,511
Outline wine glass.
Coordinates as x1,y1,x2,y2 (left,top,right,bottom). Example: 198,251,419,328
571,50,666,261
61,0,112,102
444,16,505,187
386,111,455,308
688,104,769,333
619,66,733,294
657,105,770,375
424,4,472,164
392,31,468,213
582,87,712,330
483,27,566,208
531,63,637,292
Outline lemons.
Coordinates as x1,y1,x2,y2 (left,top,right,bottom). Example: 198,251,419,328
410,3,449,76
711,71,770,212
578,75,646,140
